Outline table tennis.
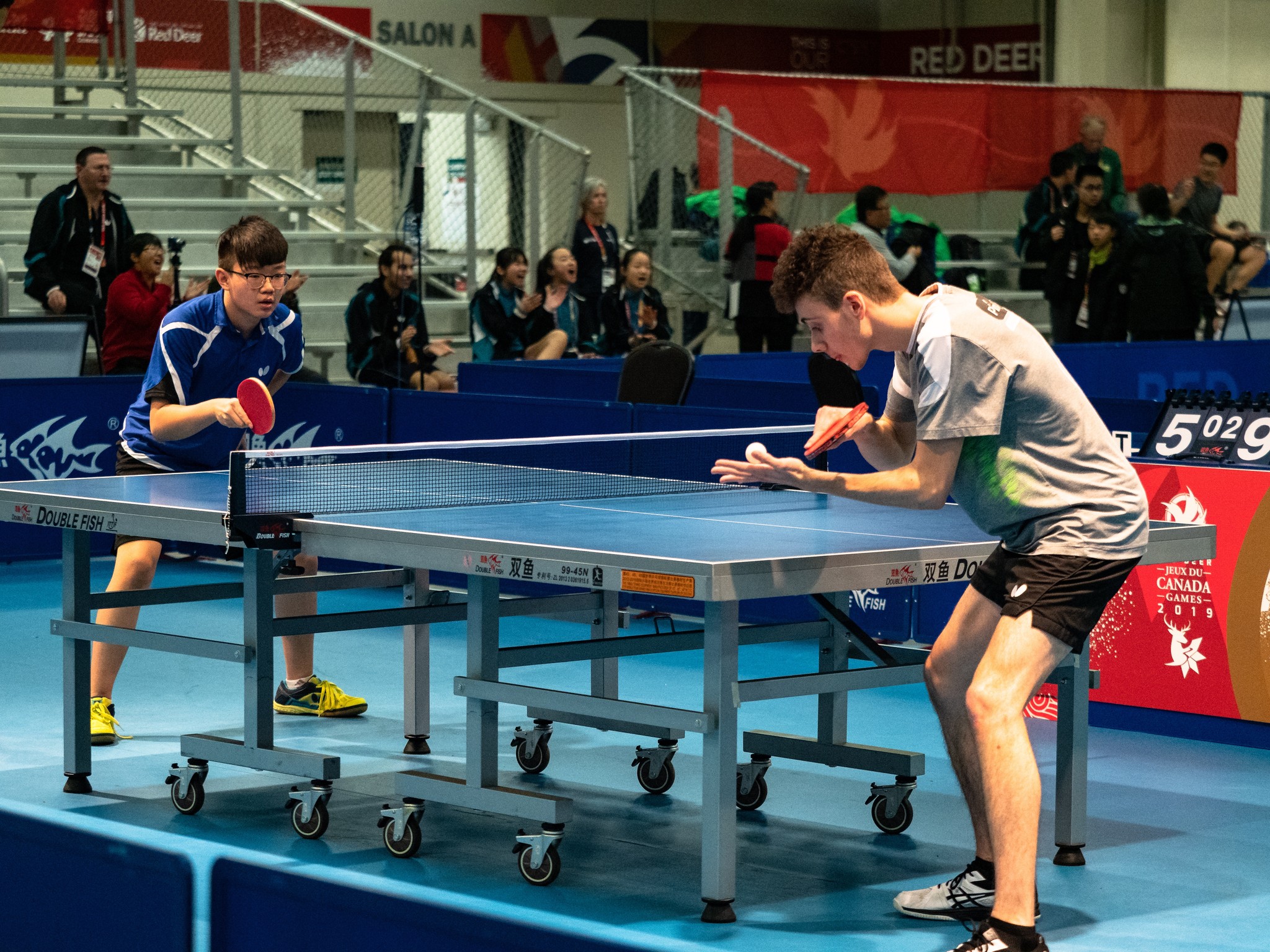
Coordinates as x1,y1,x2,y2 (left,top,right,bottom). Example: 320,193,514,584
745,441,768,465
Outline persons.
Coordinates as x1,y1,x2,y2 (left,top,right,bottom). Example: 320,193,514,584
208,269,331,384
101,233,211,378
724,179,798,353
344,243,455,391
570,176,620,356
599,248,673,356
24,146,135,376
851,185,921,281
1015,115,1266,343
463,244,568,362
533,246,607,358
711,219,1149,952
91,214,368,745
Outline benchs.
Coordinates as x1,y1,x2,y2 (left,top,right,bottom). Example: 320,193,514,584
0,77,471,385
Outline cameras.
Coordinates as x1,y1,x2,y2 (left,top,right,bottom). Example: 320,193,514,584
168,238,186,253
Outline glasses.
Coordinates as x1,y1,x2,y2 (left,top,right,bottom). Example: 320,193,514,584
227,269,292,290
142,246,166,254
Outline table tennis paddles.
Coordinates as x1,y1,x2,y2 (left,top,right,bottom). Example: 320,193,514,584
237,377,276,436
803,401,870,460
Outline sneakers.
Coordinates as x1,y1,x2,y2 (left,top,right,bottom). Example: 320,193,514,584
893,862,1040,921
942,917,1050,952
273,675,369,717
90,696,133,745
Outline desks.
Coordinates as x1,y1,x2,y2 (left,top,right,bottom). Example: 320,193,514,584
0,424,1216,925
1020,459,1270,750
